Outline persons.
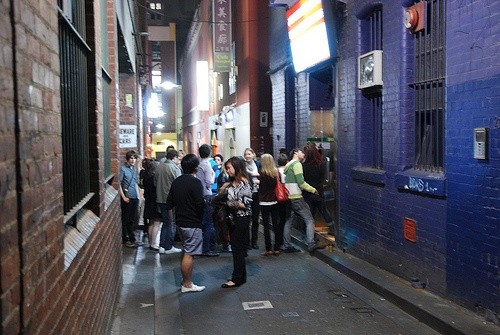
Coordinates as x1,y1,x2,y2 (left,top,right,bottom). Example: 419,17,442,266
282,149,322,254
216,154,252,290
279,148,288,154
119,149,142,251
141,143,183,256
258,153,286,256
276,154,289,247
294,140,337,237
165,153,208,295
244,147,262,250
198,136,227,290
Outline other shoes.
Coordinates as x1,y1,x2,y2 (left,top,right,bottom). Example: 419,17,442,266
262,251,272,256
252,245,259,249
247,245,252,250
221,280,241,288
178,282,206,293
125,240,144,249
150,245,159,251
283,246,301,253
166,247,181,254
274,250,283,255
159,247,165,254
328,225,335,234
306,241,319,252
203,240,233,256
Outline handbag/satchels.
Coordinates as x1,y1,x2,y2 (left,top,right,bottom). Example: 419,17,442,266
275,176,289,202
212,194,236,243
